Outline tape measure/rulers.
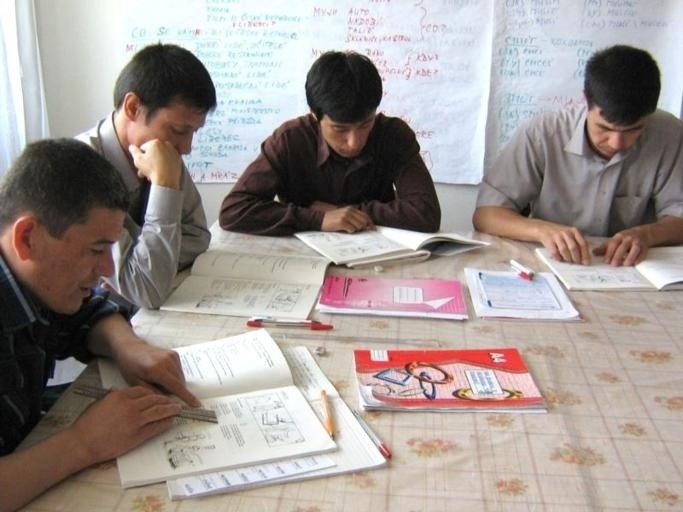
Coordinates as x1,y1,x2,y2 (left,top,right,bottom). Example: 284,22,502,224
74,382,218,424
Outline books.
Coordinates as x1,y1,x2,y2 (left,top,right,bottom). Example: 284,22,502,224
352,348,547,414
293,226,488,320
536,244,682,290
97,328,386,498
161,247,334,323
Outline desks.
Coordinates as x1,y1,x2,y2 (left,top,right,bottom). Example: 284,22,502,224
12,219,682,511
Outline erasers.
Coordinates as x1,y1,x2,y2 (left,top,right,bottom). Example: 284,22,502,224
374,265,385,273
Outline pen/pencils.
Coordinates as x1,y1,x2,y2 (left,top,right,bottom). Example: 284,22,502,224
352,409,392,458
246,319,334,329
502,259,534,280
321,390,337,440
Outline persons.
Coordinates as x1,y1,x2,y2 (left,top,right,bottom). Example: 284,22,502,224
472,45,683,266
219,51,440,235
1,138,201,512
42,45,218,412
